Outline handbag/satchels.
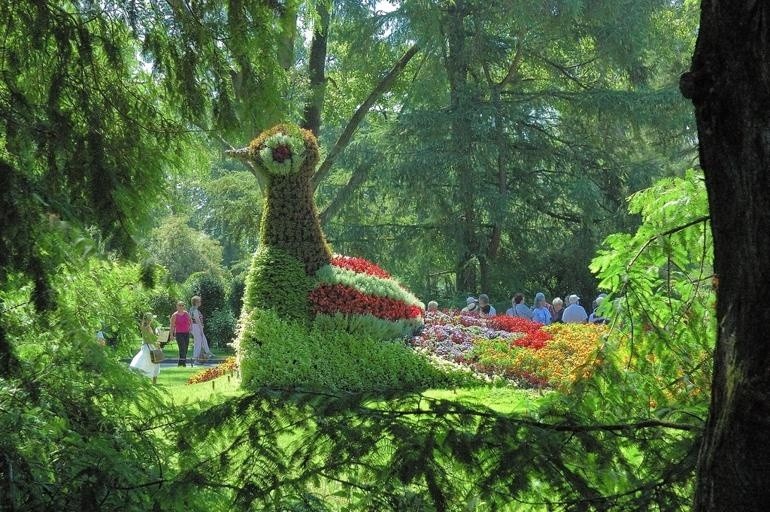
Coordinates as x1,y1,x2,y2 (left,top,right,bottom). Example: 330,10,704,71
150,349,165,364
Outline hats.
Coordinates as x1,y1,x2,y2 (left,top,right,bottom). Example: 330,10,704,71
569,295,580,303
466,297,479,312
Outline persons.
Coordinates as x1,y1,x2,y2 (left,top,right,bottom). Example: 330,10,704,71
507,292,609,326
130,311,162,386
169,295,217,367
461,293,497,320
428,300,439,312
